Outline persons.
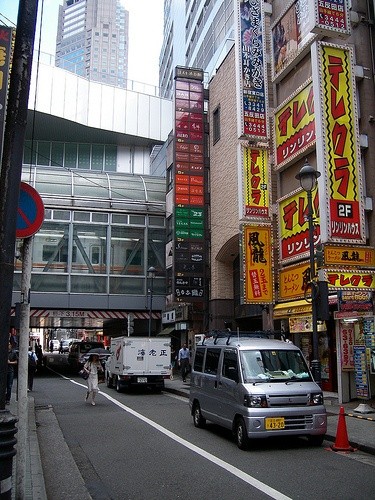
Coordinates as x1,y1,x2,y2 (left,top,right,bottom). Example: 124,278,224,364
170,343,191,382
50,341,63,354
5,327,44,405
84,354,104,405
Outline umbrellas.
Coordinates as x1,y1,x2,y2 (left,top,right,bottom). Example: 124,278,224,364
87,348,113,371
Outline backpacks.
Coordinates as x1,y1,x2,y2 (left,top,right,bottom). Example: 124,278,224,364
27,352,36,368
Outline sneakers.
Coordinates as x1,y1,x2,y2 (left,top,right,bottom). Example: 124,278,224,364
85,396,89,401
91,400,95,405
28,388,32,392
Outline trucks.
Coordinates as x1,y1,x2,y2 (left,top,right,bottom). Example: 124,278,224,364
104,337,172,394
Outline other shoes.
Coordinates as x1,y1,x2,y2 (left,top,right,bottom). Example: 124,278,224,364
5,399,10,405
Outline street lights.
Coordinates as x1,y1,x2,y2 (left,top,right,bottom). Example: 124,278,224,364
296,158,323,388
147,266,158,337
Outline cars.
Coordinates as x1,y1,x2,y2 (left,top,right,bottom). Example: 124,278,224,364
49,338,81,353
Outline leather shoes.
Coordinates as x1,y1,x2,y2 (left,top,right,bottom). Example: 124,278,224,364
183,379,186,382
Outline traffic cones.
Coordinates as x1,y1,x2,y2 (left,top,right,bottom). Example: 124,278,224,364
330,407,354,452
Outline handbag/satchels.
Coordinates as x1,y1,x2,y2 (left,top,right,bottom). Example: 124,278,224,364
79,361,90,380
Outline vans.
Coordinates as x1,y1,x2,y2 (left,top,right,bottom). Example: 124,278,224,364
188,328,327,451
68,342,107,373
